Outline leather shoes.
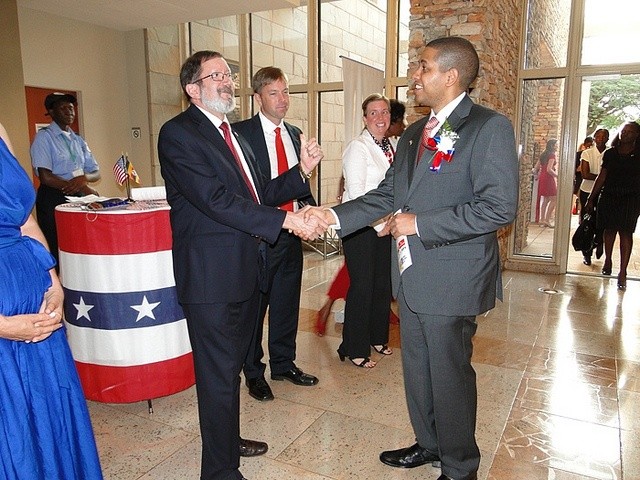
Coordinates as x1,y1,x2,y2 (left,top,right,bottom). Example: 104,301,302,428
239,438,268,457
596,243,604,259
270,366,319,386
380,444,441,468
437,474,477,480
245,375,273,400
584,256,591,265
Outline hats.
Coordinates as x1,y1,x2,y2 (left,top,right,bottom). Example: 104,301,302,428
44,92,75,108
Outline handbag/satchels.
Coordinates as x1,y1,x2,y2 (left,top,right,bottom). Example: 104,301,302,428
572,211,594,251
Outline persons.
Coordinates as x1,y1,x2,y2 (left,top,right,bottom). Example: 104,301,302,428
585,121,640,289
580,129,611,265
230,67,319,400
534,139,558,228
573,137,593,196
158,51,327,480
337,93,398,368
315,99,406,336
573,143,586,214
30,92,101,276
288,38,519,480
0,122,104,479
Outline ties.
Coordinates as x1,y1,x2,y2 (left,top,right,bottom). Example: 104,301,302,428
416,117,439,167
274,127,293,213
219,123,259,205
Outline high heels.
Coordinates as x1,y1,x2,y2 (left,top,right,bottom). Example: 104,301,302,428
602,260,611,274
538,220,545,227
370,341,392,355
544,221,554,228
617,273,627,289
337,349,375,368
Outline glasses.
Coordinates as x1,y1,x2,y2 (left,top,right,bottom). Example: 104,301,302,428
192,72,236,84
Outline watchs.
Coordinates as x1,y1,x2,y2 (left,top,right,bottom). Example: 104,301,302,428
297,162,313,179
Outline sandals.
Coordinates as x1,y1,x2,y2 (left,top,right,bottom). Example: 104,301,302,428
314,311,324,336
390,313,400,324
